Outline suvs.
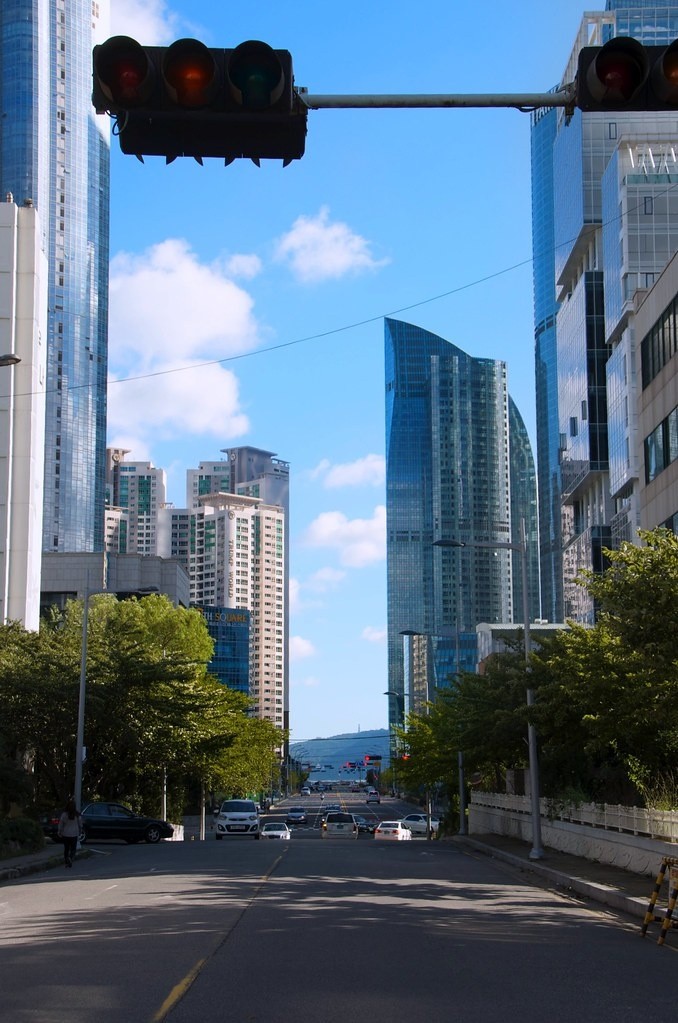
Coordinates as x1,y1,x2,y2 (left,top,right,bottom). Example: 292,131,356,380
366,790,381,804
321,812,359,840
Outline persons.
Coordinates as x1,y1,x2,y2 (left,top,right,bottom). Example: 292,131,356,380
262,799,270,815
57,801,83,868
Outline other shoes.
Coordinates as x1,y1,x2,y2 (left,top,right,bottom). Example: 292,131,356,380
65,865,68,868
67,857,72,868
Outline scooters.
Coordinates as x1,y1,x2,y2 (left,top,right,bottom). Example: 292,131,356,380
321,794,325,801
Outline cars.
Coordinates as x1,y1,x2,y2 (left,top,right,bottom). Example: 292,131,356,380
260,823,293,840
397,814,441,833
285,807,310,824
311,781,375,793
374,821,412,841
214,799,264,840
322,804,396,836
49,800,174,844
300,787,311,797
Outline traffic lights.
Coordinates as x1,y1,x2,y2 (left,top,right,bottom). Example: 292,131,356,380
341,755,382,767
92,36,293,130
402,756,409,759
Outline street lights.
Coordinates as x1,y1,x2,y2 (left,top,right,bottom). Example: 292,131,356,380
430,538,547,858
384,691,465,836
73,588,179,812
397,629,460,687
